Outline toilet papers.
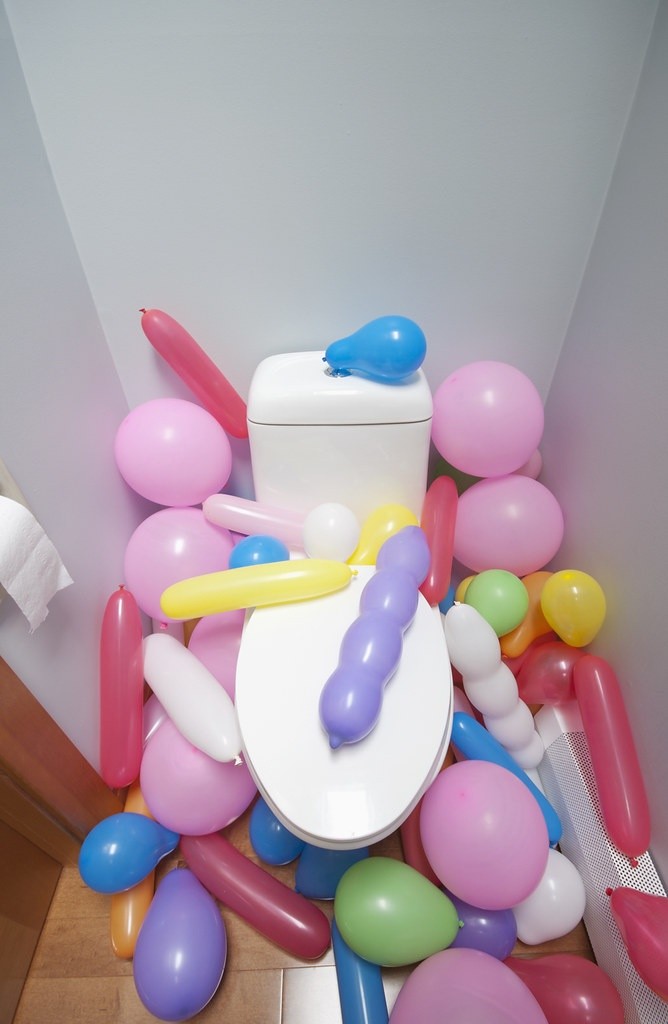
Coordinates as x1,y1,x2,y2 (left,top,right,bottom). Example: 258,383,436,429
1,495,75,635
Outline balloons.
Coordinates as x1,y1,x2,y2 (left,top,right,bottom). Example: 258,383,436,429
73,303,668,1024
320,525,432,750
419,758,550,912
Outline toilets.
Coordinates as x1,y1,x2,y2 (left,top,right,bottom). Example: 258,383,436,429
236,347,454,854
